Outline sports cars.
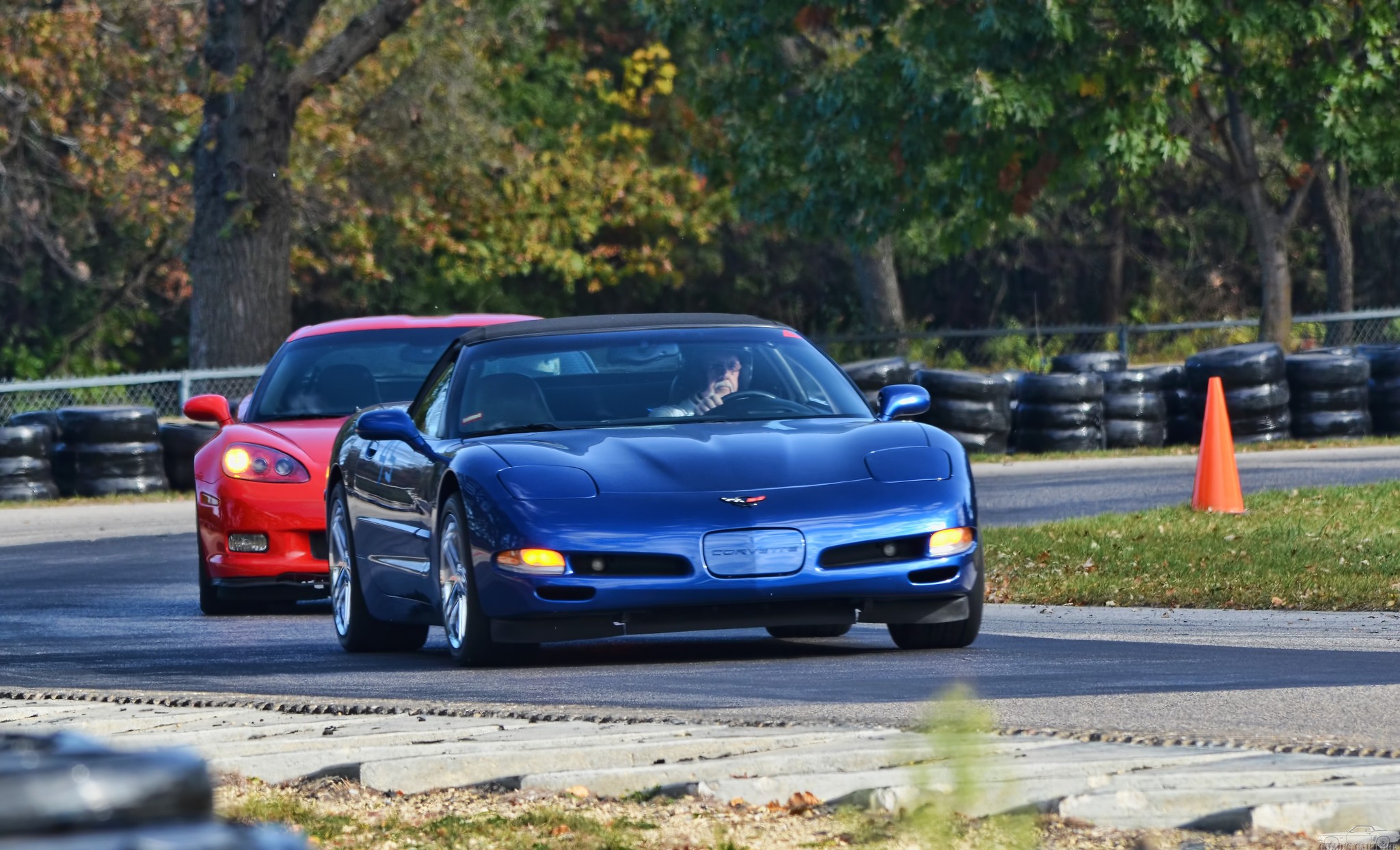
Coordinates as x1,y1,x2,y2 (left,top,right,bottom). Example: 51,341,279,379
324,305,993,649
180,316,603,617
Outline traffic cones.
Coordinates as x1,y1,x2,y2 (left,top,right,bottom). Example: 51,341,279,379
1189,375,1245,514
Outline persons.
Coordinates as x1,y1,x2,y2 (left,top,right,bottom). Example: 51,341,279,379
647,341,755,418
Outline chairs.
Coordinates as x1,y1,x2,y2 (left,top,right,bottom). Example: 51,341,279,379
311,363,379,411
472,374,553,431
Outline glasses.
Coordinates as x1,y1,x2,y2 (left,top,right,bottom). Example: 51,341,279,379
702,361,742,374
